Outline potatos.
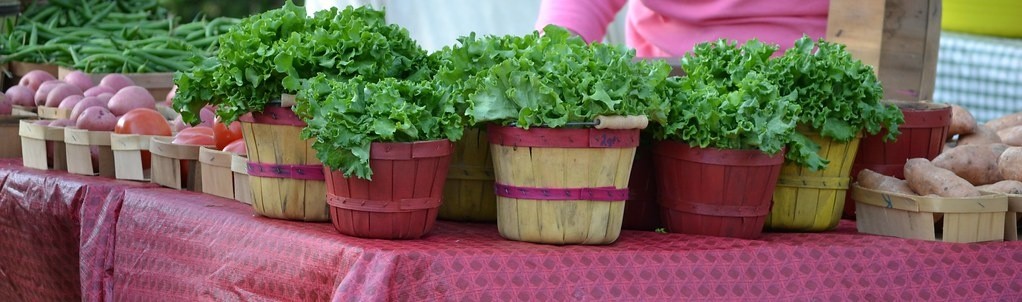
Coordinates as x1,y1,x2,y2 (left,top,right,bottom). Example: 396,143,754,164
858,102,1022,197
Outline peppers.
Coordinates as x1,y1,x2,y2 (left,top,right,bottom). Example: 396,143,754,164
0,0,241,72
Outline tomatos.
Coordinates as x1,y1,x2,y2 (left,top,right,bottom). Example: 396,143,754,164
118,109,247,184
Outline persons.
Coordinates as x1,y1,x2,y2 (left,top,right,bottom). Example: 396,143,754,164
537,0,831,63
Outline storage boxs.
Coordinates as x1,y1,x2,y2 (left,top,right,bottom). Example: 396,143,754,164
852,180,1009,245
1005,193,1022,244
1,58,251,206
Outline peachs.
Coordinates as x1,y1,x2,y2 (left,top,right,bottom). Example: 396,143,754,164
0,70,217,172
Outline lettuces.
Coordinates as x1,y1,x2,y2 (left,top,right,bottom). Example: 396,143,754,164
171,0,901,186
